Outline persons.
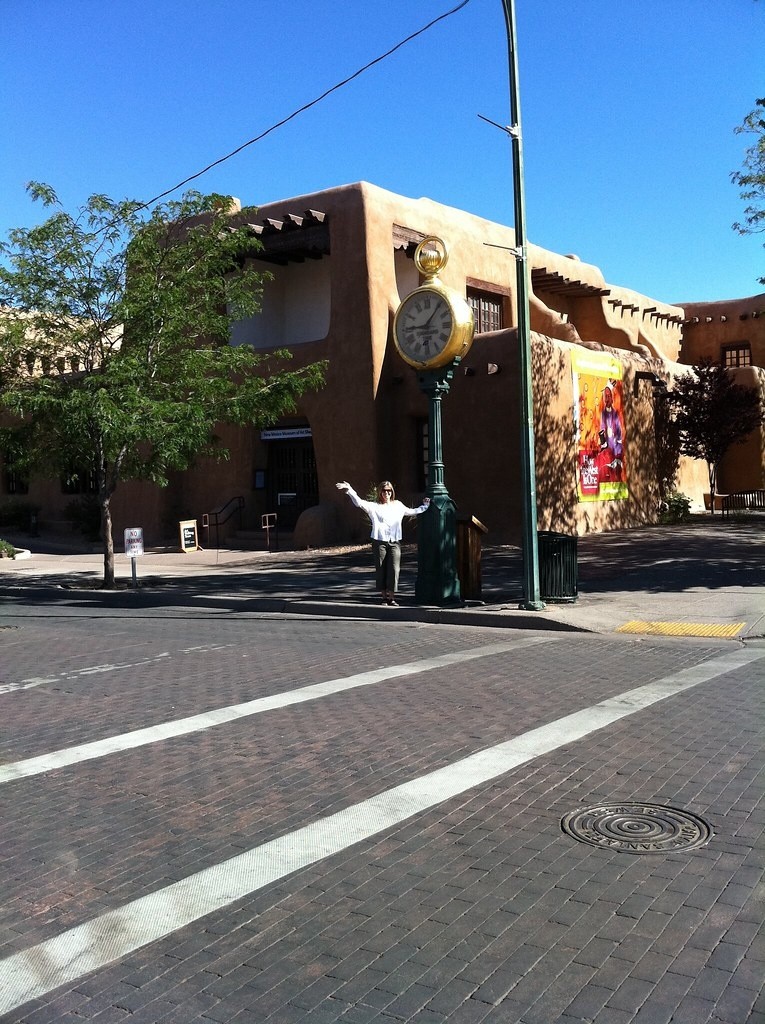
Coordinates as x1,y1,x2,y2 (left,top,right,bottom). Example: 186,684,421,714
599,386,623,456
335,480,430,607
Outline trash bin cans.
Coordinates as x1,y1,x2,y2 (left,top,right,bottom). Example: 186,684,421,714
537,531,579,604
455,515,489,599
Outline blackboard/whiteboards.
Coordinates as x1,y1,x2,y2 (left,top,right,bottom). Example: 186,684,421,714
179,519,197,553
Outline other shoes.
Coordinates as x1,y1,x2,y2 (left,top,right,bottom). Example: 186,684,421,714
388,600,399,607
381,599,388,606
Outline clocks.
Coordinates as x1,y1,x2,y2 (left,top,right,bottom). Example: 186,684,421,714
395,291,451,362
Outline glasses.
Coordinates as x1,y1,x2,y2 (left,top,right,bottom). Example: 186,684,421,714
382,489,392,493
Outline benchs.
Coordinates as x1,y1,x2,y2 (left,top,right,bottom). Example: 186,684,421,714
721,489,765,518
